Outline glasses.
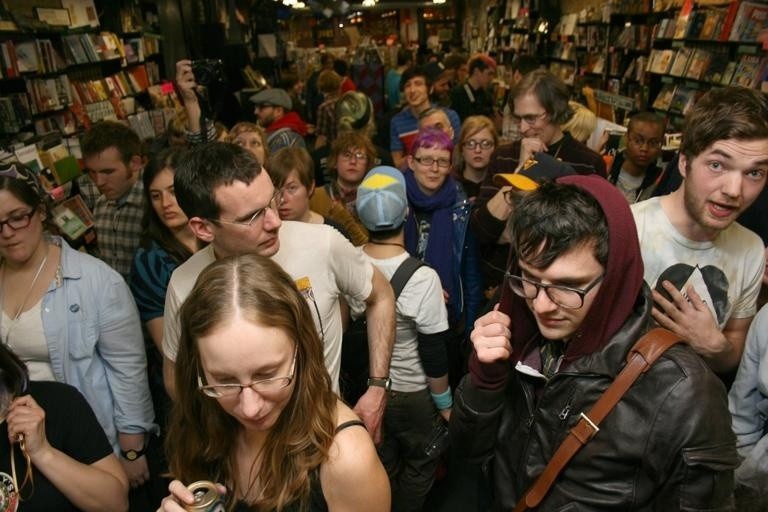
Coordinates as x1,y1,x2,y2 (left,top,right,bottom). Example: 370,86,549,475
627,132,664,150
503,255,608,310
203,187,282,231
193,339,301,401
412,155,452,168
0,203,39,233
461,138,495,150
510,111,548,125
337,150,369,162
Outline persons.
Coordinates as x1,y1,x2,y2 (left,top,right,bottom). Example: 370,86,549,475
0,1,768,512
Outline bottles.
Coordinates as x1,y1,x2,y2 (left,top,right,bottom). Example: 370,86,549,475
423,417,453,458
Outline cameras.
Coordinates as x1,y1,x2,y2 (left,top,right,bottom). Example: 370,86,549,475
188,57,226,86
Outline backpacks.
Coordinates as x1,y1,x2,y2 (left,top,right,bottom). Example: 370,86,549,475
336,312,371,407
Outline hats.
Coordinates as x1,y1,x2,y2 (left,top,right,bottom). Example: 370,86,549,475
491,151,579,196
355,165,409,233
249,87,293,111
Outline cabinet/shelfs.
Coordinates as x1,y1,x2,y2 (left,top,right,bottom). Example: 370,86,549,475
485,0,573,110
0,0,162,189
573,0,768,165
